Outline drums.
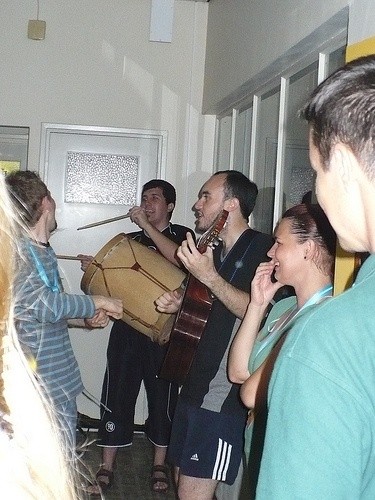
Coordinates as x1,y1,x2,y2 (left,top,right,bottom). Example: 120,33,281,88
80,232,185,345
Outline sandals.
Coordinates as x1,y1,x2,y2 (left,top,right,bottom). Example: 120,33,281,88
151,463,171,494
86,467,115,497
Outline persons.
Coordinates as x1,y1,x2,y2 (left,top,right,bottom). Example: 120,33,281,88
0,169,123,500
78,53,375,500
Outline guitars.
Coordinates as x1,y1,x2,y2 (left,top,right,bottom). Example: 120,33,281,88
167,208,230,378
